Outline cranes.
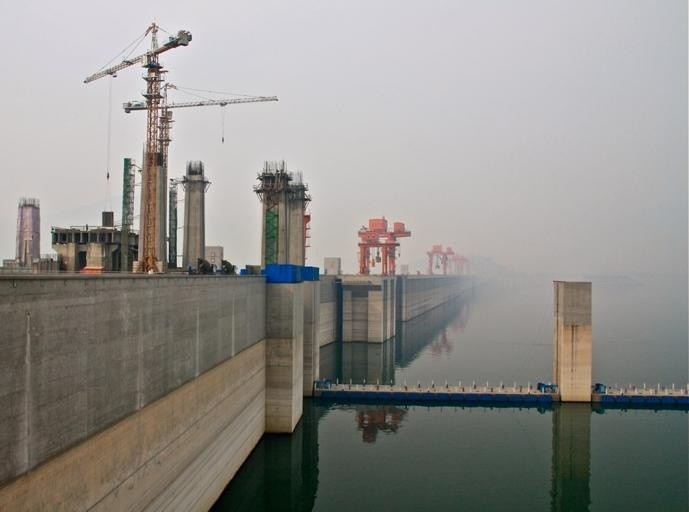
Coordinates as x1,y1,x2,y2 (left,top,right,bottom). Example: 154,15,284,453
81,14,195,276
118,81,281,269
